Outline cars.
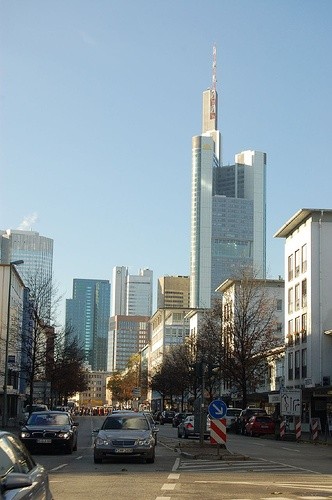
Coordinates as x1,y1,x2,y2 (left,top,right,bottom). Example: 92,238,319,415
20,404,50,425
0,430,54,500
91,412,160,464
177,415,211,439
243,415,276,437
144,413,159,446
172,412,192,428
18,410,80,455
152,411,162,421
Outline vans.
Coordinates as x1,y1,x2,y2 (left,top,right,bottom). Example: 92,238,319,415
225,407,242,433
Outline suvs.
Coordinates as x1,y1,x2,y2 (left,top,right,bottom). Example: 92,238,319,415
159,410,175,425
235,408,268,435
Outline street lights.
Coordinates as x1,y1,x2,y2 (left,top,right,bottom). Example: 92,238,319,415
2,259,25,431
